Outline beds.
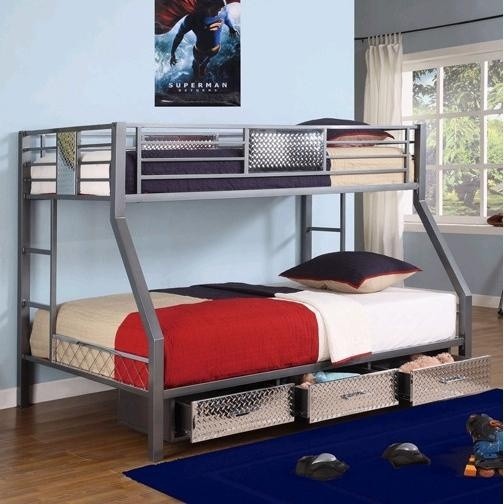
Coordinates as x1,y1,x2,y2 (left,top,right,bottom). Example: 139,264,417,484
18,122,493,464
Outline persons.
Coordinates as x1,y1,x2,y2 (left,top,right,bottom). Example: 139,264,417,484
170,0,237,77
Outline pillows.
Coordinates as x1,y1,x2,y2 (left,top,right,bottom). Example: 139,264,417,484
293,117,394,148
278,251,422,292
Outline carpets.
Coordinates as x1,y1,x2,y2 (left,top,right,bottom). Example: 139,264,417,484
122,389,503,503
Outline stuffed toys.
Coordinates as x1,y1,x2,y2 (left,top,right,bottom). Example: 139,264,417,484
400,352,453,373
464,413,503,478
